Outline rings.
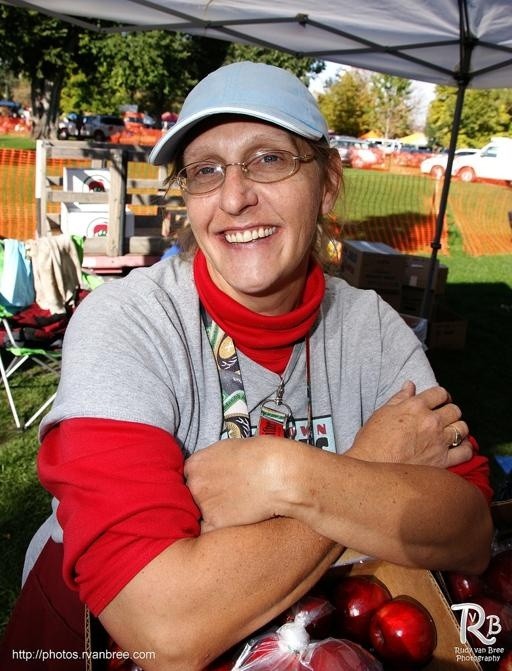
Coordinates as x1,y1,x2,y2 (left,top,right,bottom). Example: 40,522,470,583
447,423,464,449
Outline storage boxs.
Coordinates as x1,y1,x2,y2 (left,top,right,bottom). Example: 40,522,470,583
61,167,134,239
341,241,467,351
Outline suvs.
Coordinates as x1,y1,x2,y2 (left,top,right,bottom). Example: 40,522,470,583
58,112,125,142
332,140,384,169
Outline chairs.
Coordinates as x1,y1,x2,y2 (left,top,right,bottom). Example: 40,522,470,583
1,235,102,431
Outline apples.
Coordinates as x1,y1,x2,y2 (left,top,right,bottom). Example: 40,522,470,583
219,548,436,671
448,550,512,671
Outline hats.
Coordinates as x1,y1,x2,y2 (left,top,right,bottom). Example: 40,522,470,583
150,61,331,164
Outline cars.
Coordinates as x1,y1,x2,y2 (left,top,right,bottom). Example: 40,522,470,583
451,138,512,185
419,148,481,179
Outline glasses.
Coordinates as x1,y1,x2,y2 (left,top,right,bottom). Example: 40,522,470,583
171,148,318,194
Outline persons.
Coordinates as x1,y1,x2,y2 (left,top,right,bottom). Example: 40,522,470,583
75,109,86,140
21,102,33,128
1,58,496,671
